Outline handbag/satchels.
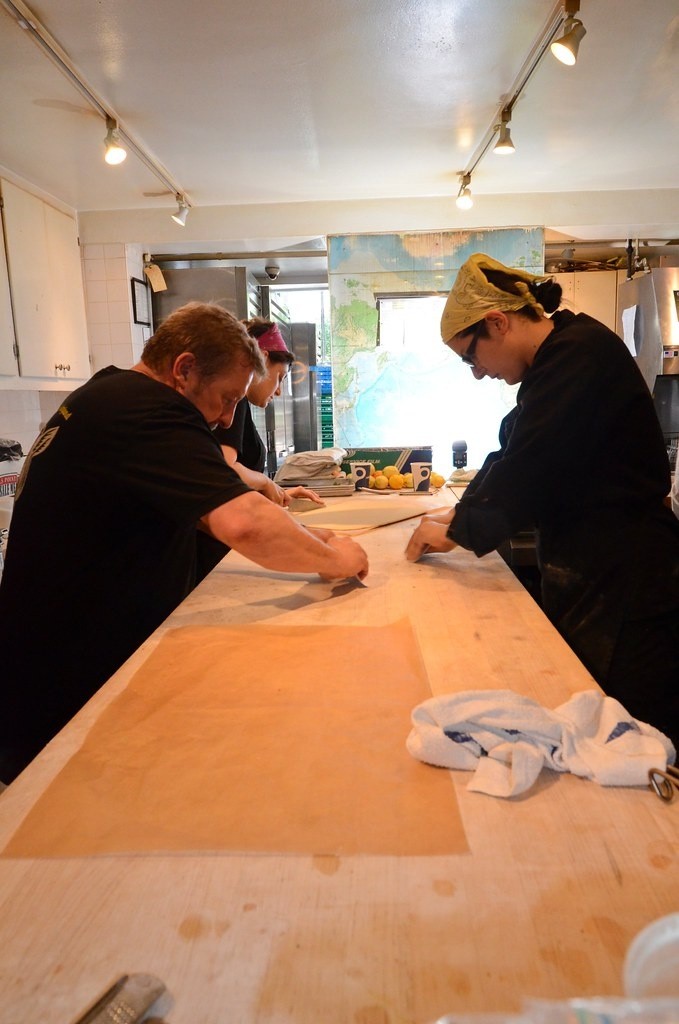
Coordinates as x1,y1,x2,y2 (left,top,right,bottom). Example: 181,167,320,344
0,438,22,461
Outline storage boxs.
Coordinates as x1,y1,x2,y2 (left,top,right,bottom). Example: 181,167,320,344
320,367,333,449
341,446,432,486
0,456,27,498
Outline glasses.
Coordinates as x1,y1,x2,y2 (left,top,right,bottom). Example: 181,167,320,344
462,332,479,366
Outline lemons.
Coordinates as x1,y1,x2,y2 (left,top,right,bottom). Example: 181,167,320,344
347,464,446,489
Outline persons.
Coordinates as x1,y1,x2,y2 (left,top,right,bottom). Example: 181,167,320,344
1,305,370,788
405,251,679,728
198,314,329,578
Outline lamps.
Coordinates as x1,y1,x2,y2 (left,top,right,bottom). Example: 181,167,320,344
551,0,586,66
491,109,515,155
456,175,472,210
103,118,127,165
172,195,189,227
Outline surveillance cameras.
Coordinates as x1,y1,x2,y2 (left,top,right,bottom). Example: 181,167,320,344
265,266,279,280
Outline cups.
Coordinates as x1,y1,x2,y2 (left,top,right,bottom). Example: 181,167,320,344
410,462,432,492
350,463,371,491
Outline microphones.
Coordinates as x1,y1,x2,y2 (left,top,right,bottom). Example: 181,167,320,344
451,440,467,469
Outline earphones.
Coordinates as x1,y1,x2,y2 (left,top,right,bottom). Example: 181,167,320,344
180,364,192,379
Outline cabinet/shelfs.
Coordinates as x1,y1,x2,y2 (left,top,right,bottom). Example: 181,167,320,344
544,271,617,333
0,165,92,391
